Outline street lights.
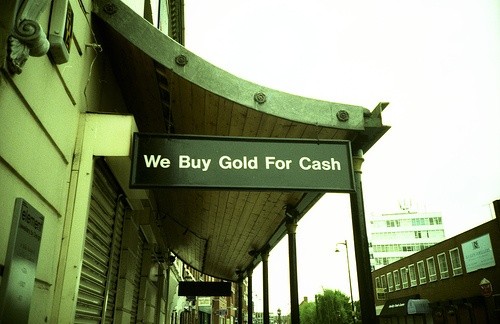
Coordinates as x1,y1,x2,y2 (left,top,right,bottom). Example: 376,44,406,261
335,239,357,324
